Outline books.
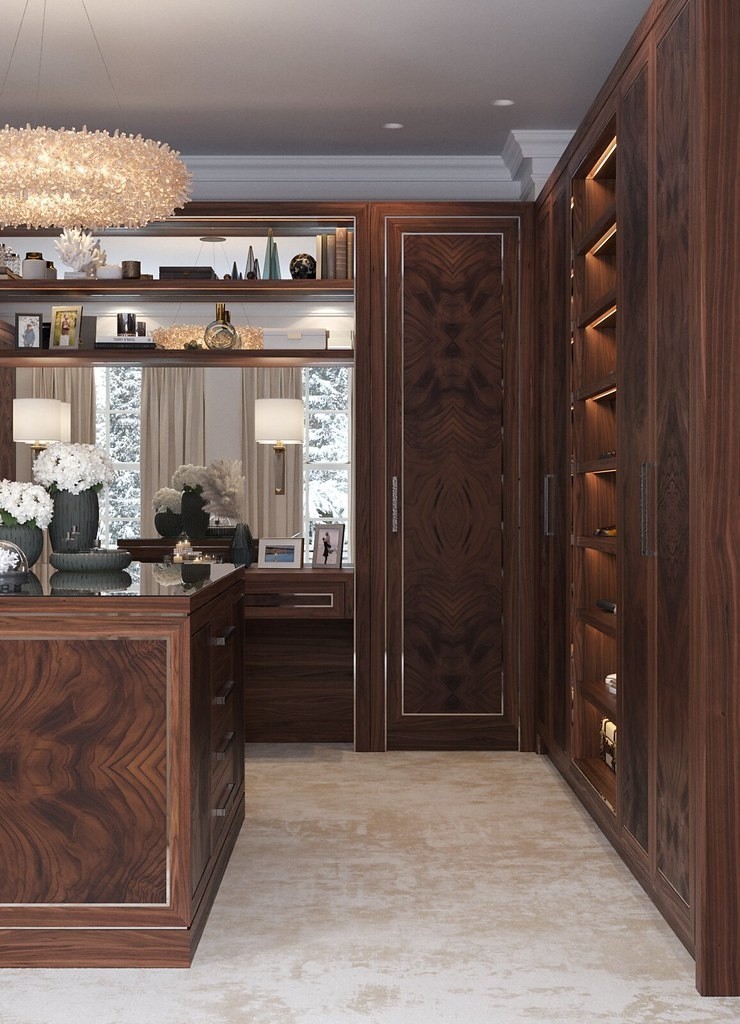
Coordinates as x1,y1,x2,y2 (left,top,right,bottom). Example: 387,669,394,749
335,228,346,279
316,235,321,279
347,232,354,280
327,235,335,279
322,234,327,279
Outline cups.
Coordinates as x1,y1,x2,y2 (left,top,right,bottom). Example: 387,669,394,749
117,313,136,337
121,260,141,279
138,322,146,337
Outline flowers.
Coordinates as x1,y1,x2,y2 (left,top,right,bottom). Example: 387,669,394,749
0,444,114,529
153,464,211,515
152,562,183,586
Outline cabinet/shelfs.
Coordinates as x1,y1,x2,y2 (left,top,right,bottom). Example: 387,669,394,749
0,567,355,972
548,0,740,998
0,203,361,367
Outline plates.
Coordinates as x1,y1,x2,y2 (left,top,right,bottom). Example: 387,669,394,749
49,549,132,573
48,571,132,590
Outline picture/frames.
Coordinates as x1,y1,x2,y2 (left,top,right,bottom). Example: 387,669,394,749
49,305,83,350
259,523,345,568
14,313,44,351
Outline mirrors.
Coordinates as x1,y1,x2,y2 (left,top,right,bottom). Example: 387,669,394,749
0,226,356,351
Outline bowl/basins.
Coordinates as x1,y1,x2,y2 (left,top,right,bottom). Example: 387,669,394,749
96,269,122,279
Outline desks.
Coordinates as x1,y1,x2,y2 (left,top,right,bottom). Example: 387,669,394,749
117,537,259,566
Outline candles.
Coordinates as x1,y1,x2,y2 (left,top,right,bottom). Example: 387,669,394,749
173,539,211,564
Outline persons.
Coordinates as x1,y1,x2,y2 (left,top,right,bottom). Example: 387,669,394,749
323,531,334,565
23,323,36,347
275,549,277,562
59,314,71,345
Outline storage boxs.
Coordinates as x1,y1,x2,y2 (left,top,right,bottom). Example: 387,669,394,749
263,328,329,350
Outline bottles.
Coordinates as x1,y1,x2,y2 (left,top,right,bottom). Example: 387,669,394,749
45,261,57,279
228,523,256,568
203,303,236,350
21,252,46,280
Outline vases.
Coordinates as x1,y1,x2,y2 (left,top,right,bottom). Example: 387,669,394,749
155,492,209,537
0,483,99,568
231,522,256,567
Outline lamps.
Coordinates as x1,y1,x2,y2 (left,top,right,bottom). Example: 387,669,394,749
0,0,193,233
253,399,304,495
12,397,71,462
150,237,264,350
